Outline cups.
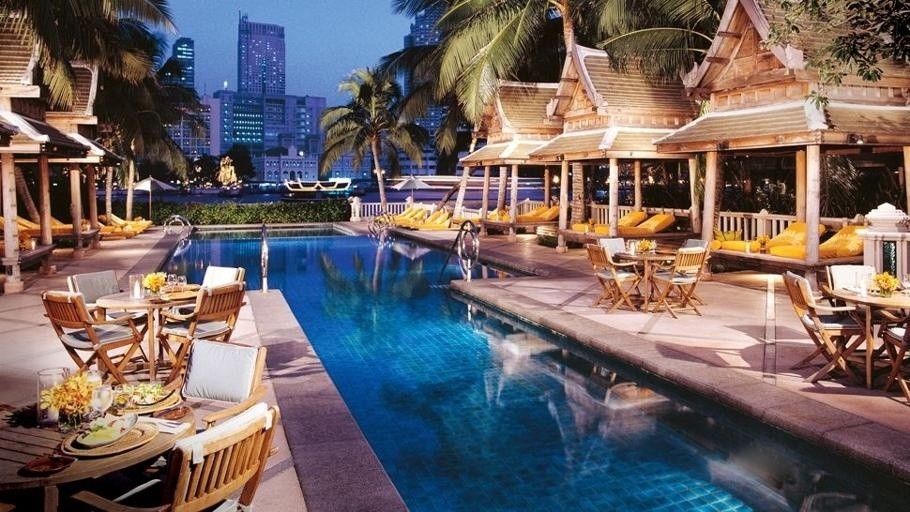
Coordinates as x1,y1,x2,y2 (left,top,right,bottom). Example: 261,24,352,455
128,274,144,299
903,273,910,298
854,270,872,300
36,367,71,428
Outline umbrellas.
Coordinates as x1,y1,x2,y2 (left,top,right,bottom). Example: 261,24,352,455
133,174,178,219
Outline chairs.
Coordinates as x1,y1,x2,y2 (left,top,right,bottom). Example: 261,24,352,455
0,207,152,269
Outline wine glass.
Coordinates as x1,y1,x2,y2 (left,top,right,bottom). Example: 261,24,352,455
81,370,114,417
167,274,187,294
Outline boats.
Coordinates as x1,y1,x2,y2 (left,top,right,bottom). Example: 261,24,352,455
190,186,243,197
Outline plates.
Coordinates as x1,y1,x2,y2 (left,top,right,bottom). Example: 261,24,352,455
186,284,200,291
108,390,182,416
164,290,199,301
61,421,161,456
75,412,138,449
